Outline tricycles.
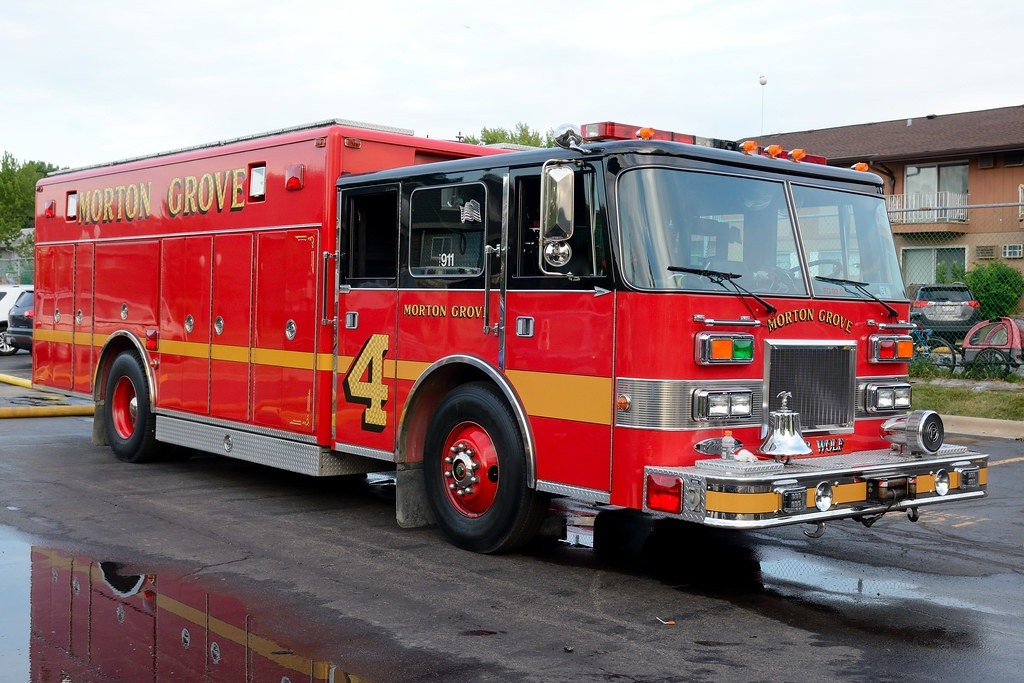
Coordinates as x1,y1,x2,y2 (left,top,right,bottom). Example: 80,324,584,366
910,312,1024,379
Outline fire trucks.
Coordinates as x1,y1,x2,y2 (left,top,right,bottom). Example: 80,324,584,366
29,547,363,683
32,121,989,555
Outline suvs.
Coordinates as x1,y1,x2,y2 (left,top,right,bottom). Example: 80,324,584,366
6,289,33,354
36,290,188,360
906,281,981,345
0,284,34,355
